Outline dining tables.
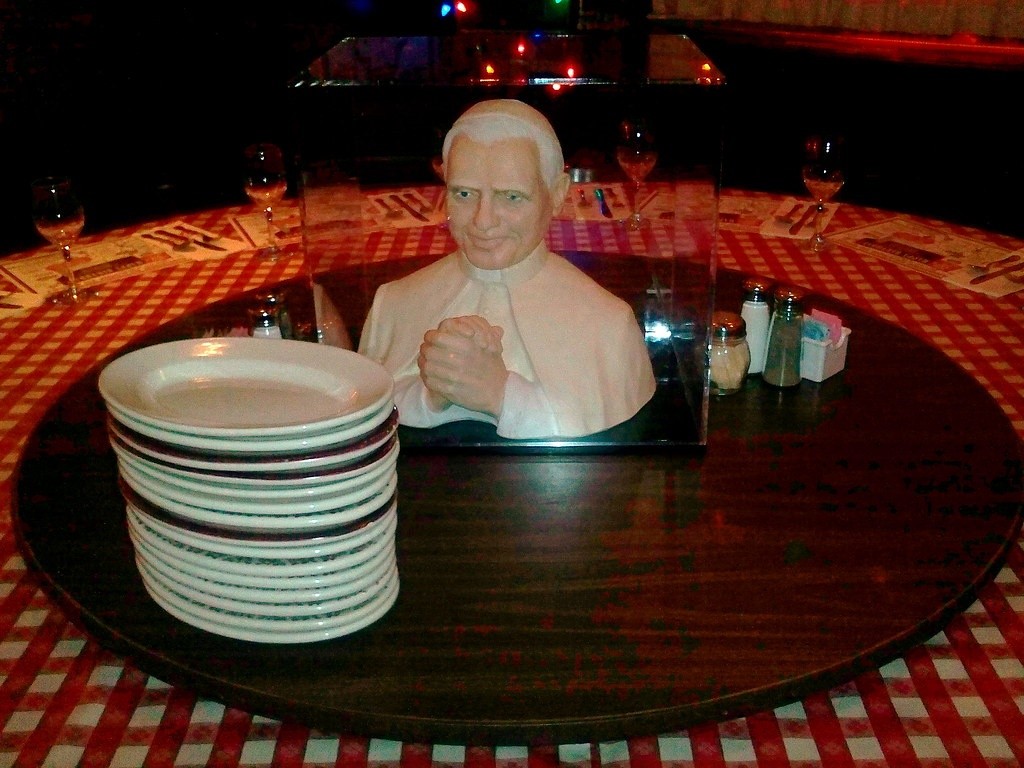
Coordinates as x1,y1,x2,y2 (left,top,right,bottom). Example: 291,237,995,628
1,181,1022,767
290,34,726,92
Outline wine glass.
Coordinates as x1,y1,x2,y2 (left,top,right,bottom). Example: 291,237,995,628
243,143,294,262
801,138,844,253
31,175,99,310
617,120,657,230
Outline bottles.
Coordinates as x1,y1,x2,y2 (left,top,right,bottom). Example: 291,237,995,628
761,288,803,385
694,311,751,396
248,311,283,339
266,294,294,340
740,277,773,374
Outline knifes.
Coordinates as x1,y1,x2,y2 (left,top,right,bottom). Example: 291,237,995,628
789,205,817,235
154,230,227,252
595,188,612,218
0,303,22,309
969,261,1024,285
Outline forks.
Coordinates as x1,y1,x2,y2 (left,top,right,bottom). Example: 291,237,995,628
807,211,819,227
1004,273,1024,284
173,225,222,242
606,188,624,207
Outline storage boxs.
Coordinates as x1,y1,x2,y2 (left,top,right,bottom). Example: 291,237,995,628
800,325,851,383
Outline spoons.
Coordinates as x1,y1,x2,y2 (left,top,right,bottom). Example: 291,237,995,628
576,189,591,209
775,204,804,224
141,233,191,251
971,255,1020,273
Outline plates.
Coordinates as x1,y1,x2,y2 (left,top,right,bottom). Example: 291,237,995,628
99,336,401,643
